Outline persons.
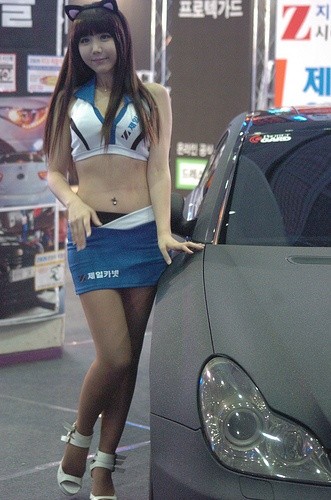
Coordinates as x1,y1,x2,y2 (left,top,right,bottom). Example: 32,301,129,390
44,2,206,500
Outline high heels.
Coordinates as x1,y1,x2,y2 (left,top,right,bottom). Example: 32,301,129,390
56,421,95,497
90,448,127,500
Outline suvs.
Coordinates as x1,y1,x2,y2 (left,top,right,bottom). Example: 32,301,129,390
148,103,331,500
